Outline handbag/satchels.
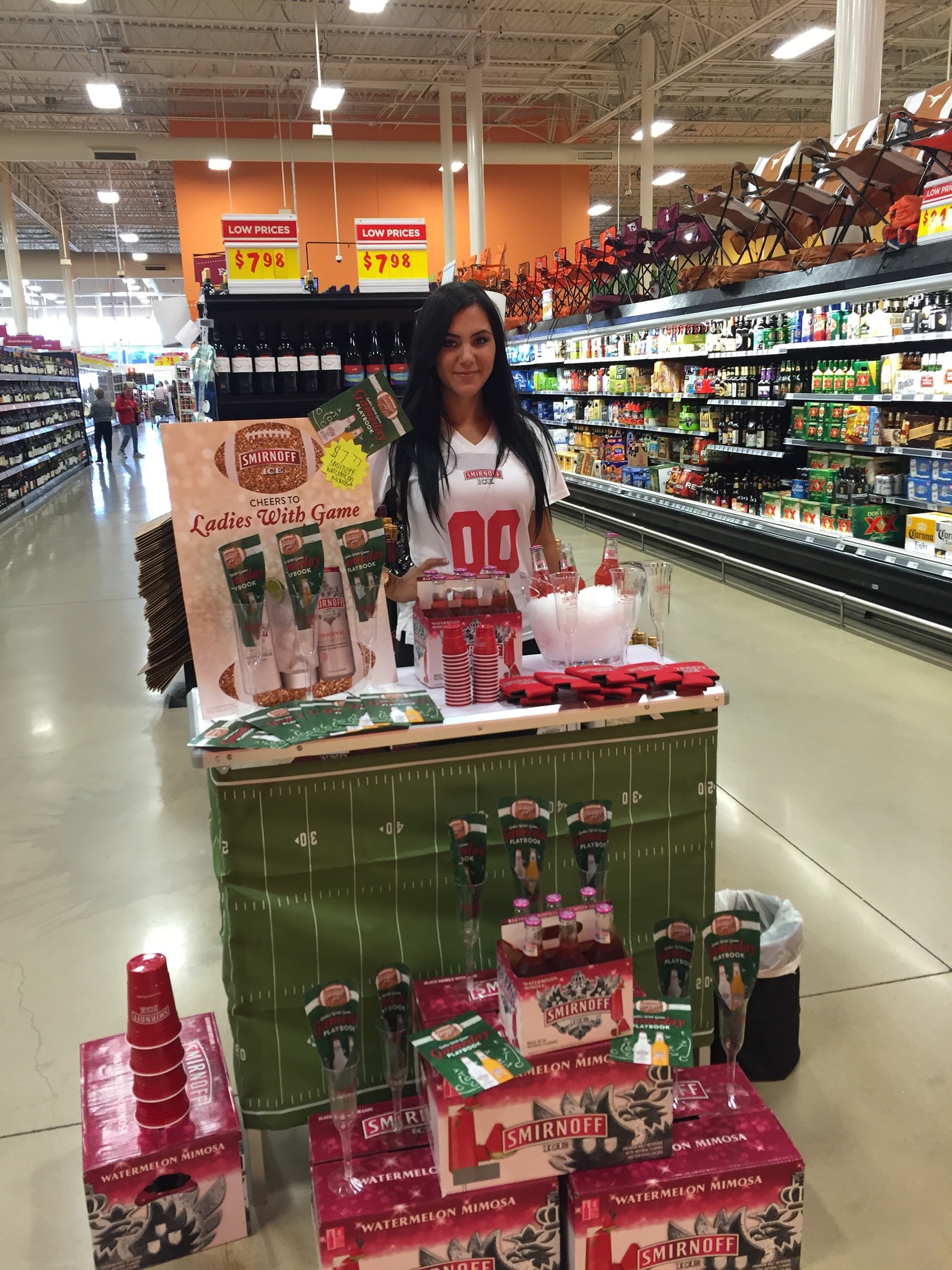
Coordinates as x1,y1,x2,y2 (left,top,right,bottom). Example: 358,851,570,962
134,411,139,426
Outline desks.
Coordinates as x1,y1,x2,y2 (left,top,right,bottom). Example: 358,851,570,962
187,646,731,1129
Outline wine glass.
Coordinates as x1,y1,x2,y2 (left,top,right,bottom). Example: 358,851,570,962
713,983,751,1110
549,562,673,676
373,1012,418,1149
453,872,543,1003
320,1051,370,1197
671,1065,693,1114
229,582,378,709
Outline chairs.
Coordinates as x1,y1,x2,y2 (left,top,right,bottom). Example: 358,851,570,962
428,79,952,327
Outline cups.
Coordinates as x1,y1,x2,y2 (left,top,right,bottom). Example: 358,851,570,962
125,951,191,1129
576,866,609,904
440,621,499,707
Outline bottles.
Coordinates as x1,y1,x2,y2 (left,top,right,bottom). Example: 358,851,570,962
423,565,514,618
666,969,681,999
250,705,426,743
511,886,625,979
352,572,376,599
376,504,402,577
331,1034,356,1073
0,349,88,510
634,1028,670,1066
196,267,410,395
545,287,952,516
717,961,745,1012
301,579,314,628
246,591,260,646
460,1049,514,1090
317,414,365,448
514,848,598,893
527,532,658,650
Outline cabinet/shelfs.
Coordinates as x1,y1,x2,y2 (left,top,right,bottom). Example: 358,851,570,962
479,248,952,670
0,338,197,530
201,288,440,422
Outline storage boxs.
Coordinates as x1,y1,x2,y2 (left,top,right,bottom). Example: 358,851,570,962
504,312,952,563
413,593,522,688
305,900,805,1270
79,1011,251,1270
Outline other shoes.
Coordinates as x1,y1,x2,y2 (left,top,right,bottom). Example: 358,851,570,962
133,452,145,457
118,450,127,458
95,459,103,463
108,459,112,463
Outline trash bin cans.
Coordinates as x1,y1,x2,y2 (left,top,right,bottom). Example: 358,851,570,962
714,889,803,1083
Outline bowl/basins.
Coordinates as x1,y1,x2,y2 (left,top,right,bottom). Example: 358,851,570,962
519,561,647,671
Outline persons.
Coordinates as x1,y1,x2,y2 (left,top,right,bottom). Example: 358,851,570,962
114,382,145,458
85,387,114,463
153,380,180,421
361,278,571,669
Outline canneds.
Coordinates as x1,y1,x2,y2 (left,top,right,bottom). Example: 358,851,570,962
315,566,356,681
874,473,910,495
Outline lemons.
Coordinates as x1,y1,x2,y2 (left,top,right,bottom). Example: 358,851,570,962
264,578,286,603
